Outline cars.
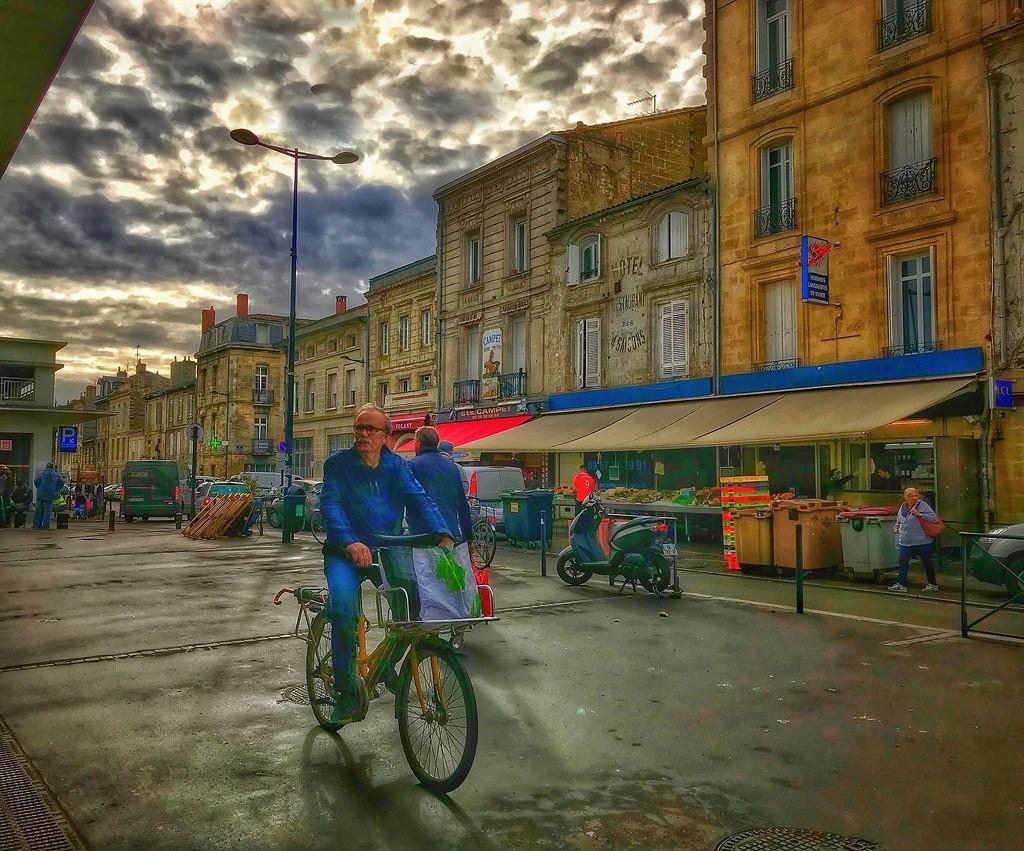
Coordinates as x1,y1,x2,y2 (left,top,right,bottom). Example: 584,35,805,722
94,483,122,501
180,472,304,526
268,478,324,529
969,524,1024,604
65,482,77,492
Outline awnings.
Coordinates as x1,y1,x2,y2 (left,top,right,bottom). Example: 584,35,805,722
395,414,534,452
454,377,977,453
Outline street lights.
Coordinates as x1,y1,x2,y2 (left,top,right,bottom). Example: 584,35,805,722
208,352,231,481
228,127,360,543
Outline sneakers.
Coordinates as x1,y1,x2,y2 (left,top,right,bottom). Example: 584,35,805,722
921,583,939,592
888,583,907,593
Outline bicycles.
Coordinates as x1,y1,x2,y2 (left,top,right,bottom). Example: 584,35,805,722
306,491,328,544
465,495,498,570
272,533,502,795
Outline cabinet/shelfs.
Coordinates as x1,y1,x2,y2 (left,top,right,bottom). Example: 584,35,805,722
894,453,934,492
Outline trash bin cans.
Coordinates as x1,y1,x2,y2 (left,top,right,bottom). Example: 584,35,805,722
730,504,773,574
502,489,556,546
836,504,908,579
771,498,847,578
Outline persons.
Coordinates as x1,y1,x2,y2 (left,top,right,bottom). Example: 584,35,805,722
0,464,32,526
821,469,864,494
888,488,938,591
320,403,453,725
75,477,104,495
572,465,595,516
31,462,64,528
407,426,473,554
879,465,899,488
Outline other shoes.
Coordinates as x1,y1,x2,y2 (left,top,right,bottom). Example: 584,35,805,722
330,694,354,723
381,667,401,695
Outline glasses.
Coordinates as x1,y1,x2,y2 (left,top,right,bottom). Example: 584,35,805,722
353,424,386,436
835,471,842,475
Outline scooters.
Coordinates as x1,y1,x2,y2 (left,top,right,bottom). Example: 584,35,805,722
551,469,678,596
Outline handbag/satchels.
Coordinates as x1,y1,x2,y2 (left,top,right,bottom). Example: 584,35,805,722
379,541,484,621
468,546,496,616
915,501,946,538
86,496,92,510
51,495,66,506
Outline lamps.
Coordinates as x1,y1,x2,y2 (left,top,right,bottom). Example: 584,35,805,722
773,443,781,451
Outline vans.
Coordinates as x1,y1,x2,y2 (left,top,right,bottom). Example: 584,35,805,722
120,460,192,522
458,466,526,534
